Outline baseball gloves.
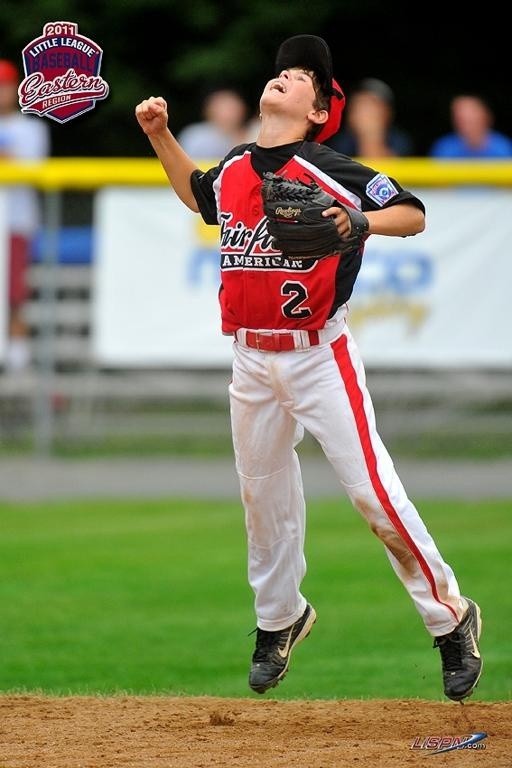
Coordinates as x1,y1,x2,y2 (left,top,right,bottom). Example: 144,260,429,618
259,171,369,262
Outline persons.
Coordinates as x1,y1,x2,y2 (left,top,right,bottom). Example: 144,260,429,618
431,93,511,158
327,79,417,156
0,58,53,377
178,85,260,161
133,58,484,701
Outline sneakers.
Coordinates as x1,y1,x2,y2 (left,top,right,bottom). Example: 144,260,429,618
433,595,484,704
247,602,318,694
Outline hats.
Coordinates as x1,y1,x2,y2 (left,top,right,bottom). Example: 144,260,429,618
277,34,346,143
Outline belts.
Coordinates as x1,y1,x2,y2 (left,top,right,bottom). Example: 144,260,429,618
233,317,348,351
